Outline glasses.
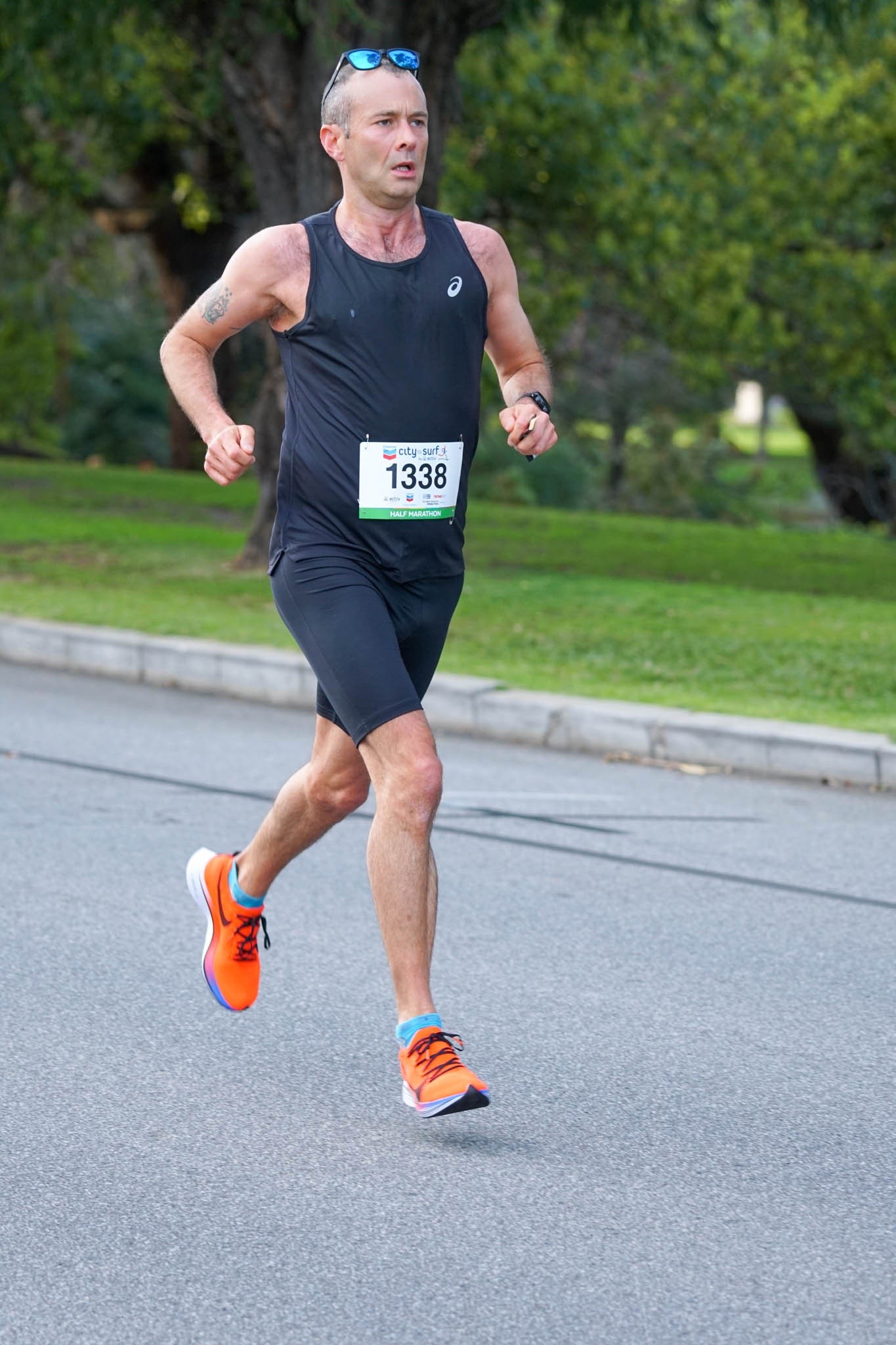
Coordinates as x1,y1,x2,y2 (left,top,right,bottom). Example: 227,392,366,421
320,47,422,110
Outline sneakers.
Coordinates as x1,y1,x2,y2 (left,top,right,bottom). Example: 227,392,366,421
398,1025,493,1120
185,848,273,1011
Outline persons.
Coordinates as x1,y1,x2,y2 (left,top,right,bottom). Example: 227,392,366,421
157,47,560,1120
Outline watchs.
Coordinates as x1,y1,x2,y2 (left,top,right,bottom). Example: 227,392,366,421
515,391,552,415
